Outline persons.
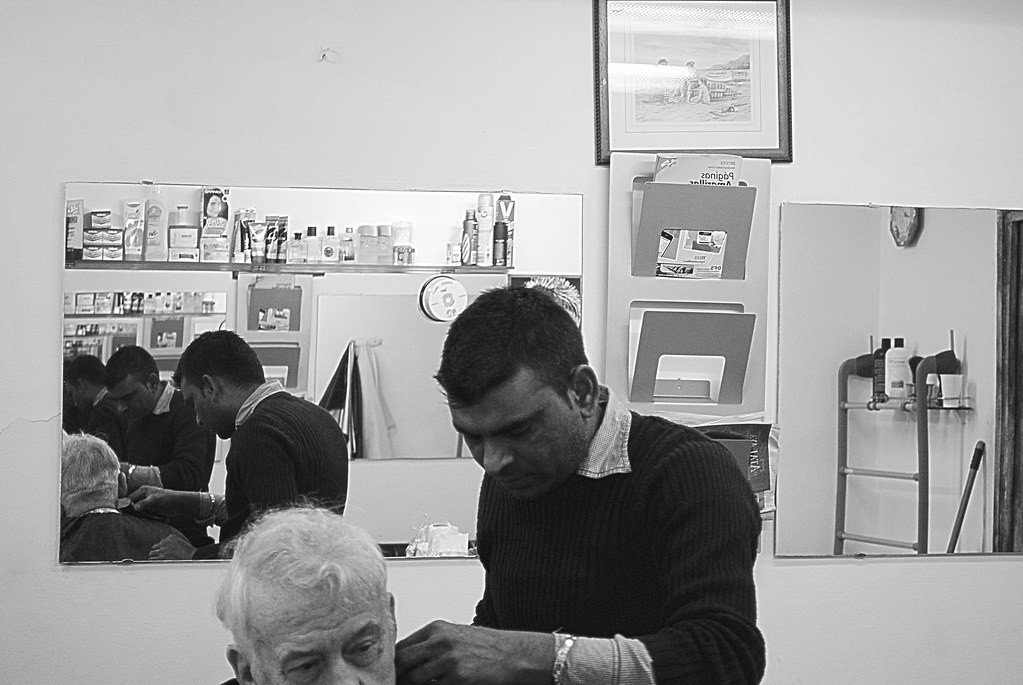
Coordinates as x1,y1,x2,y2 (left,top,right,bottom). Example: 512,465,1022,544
212,506,399,685
61,330,349,564
394,287,765,685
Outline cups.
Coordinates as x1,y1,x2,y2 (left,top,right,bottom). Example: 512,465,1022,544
940,374,964,407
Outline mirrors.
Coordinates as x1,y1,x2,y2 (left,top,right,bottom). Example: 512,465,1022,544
772,201,1023,559
57,180,584,567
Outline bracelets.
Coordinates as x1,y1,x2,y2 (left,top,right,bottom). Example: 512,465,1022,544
205,492,217,518
126,464,136,491
553,634,576,685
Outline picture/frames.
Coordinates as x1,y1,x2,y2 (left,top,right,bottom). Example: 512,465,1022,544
592,0,794,167
507,273,583,333
